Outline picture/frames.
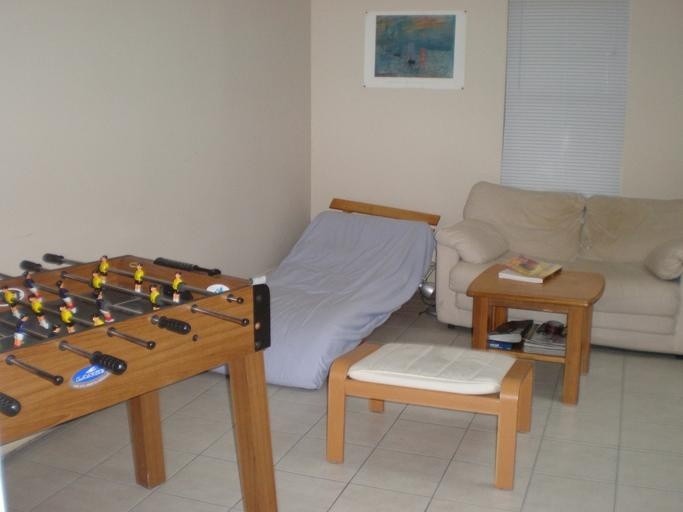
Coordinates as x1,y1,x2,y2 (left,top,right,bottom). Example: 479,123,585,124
362,8,466,90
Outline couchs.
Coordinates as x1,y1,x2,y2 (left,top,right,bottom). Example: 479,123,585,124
433,181,683,360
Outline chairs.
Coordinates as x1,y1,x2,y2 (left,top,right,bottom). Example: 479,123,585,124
207,197,440,390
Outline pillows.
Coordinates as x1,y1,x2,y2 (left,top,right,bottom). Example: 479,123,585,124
434,218,509,264
643,238,683,281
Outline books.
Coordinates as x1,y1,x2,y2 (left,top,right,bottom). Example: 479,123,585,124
487,319,565,359
497,253,562,283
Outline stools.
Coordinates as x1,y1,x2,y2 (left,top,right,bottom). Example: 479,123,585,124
466,264,606,406
326,342,536,490
